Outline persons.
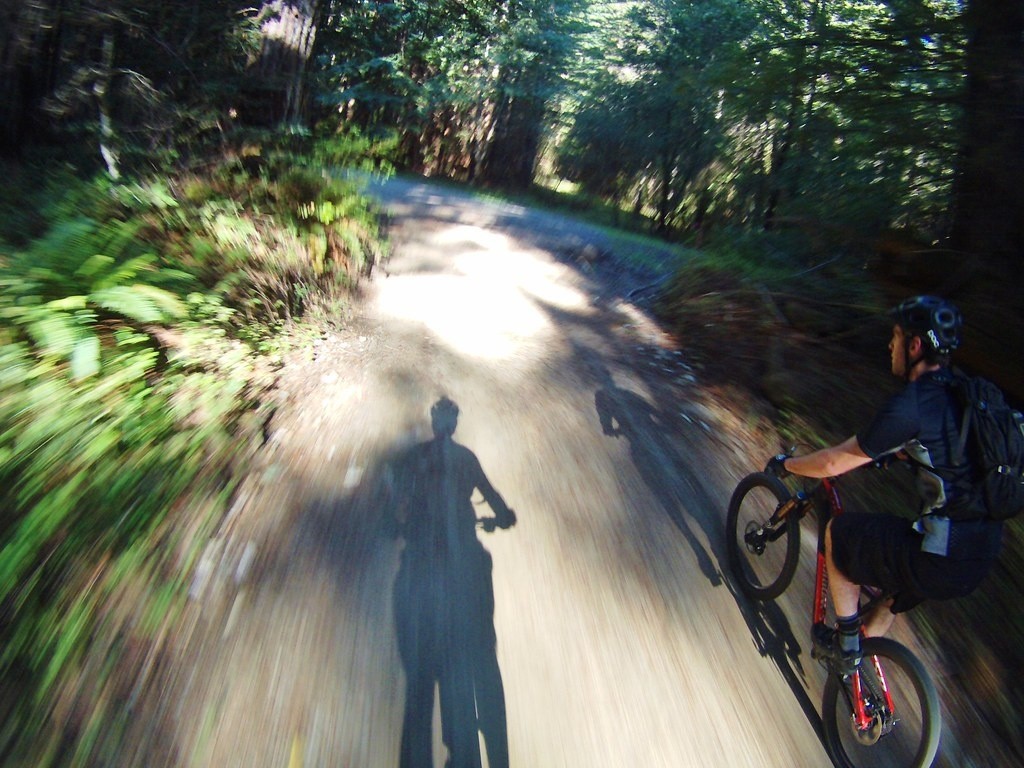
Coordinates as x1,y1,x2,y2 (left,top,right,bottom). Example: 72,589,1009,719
764,296,1005,676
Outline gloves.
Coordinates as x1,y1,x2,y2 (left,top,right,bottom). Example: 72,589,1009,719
767,454,793,477
873,452,900,470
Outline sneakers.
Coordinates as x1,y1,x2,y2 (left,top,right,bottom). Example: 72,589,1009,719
810,621,864,674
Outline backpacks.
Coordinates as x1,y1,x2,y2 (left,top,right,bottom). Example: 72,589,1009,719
901,374,1024,522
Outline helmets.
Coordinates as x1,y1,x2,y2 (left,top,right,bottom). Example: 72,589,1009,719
887,295,962,353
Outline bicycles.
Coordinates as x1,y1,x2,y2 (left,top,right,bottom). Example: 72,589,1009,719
726,442,942,768
